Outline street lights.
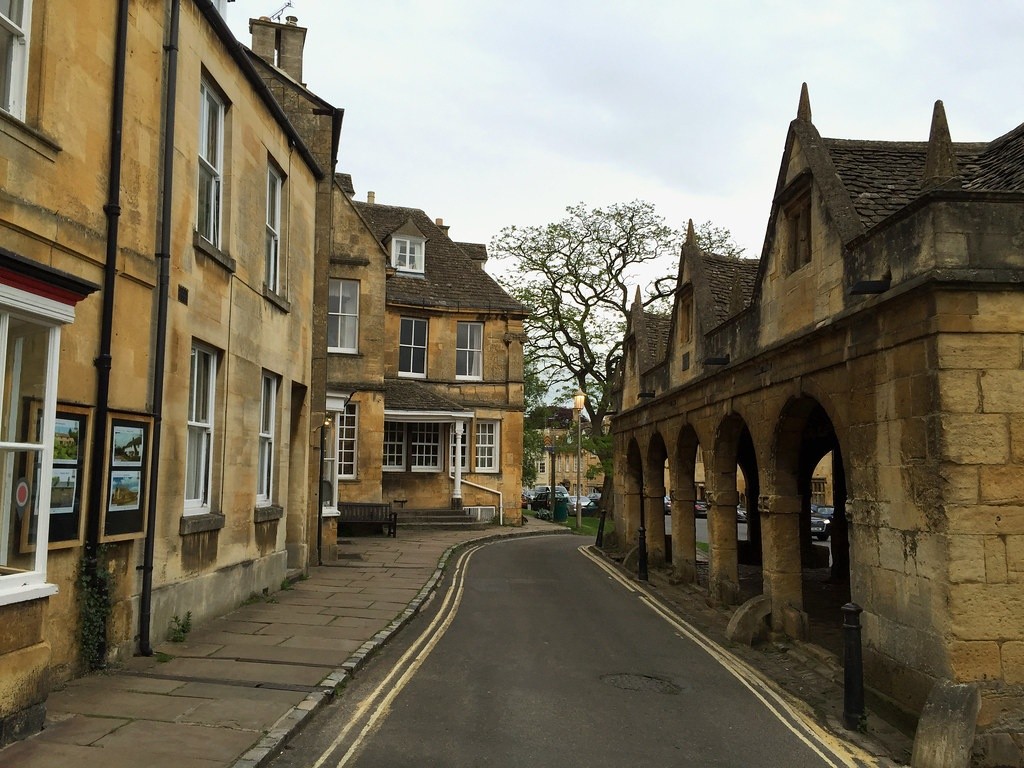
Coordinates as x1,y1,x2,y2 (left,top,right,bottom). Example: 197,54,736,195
574,387,587,529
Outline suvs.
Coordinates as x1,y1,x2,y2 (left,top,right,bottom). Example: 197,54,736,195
810,504,834,540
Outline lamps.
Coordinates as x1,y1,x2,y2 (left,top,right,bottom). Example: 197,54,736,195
314,414,332,433
848,280,891,296
637,390,656,400
702,357,730,365
602,408,619,416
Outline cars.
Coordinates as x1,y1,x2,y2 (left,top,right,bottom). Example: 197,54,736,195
695,502,708,518
567,496,596,516
587,493,602,506
664,497,671,514
737,507,747,522
531,491,569,512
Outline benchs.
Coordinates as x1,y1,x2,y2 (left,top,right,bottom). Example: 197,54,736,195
336,502,398,538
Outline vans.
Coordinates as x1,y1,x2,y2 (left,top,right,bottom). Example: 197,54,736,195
535,486,570,503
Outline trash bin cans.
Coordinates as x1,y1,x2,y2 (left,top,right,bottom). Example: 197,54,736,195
555,497,569,523
